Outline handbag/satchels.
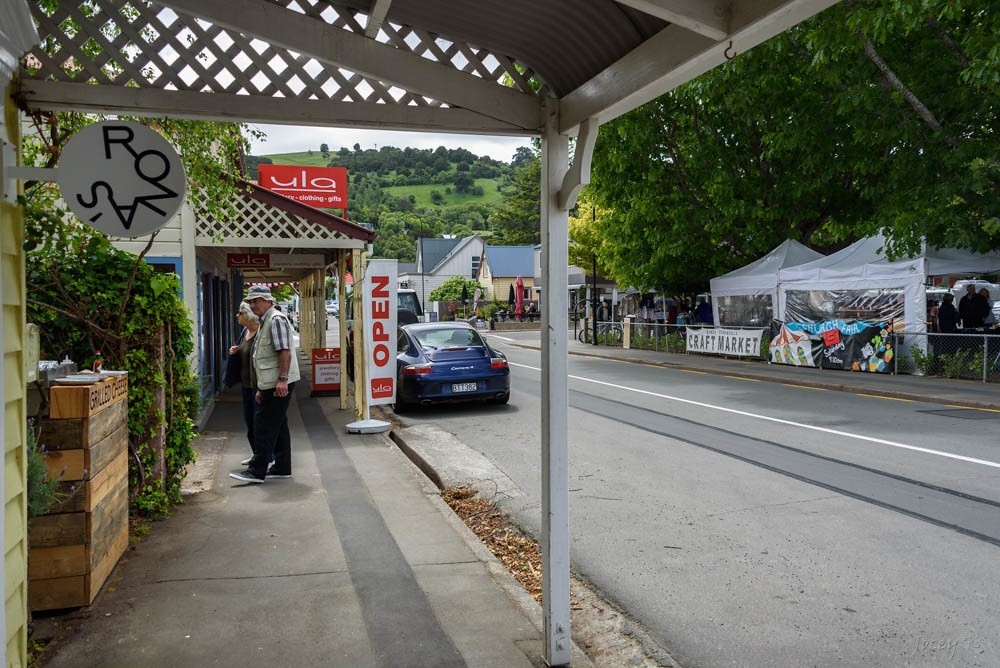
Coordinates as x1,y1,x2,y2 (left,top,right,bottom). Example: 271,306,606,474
221,325,249,387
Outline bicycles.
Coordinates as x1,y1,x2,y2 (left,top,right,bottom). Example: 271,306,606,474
578,318,623,344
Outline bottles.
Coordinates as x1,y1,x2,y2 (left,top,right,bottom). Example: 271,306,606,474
93,351,102,374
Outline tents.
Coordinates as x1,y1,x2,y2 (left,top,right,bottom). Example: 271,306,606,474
709,238,828,328
777,226,1000,376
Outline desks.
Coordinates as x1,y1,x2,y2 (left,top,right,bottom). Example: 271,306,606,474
493,313,541,322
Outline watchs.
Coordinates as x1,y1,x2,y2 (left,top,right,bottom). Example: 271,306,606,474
279,376,288,381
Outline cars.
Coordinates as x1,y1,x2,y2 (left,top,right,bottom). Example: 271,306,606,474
285,298,346,322
393,320,511,415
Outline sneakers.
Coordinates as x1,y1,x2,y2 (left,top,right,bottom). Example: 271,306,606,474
228,464,293,483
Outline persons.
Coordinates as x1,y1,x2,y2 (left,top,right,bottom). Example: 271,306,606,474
526,302,536,314
667,296,714,337
597,298,612,334
229,301,275,470
937,293,961,331
958,284,991,329
978,288,996,324
229,284,301,482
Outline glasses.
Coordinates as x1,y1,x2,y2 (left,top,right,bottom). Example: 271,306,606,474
237,310,246,315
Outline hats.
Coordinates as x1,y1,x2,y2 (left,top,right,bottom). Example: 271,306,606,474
242,286,277,301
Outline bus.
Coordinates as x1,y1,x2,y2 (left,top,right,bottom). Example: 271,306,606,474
339,288,425,357
293,292,329,333
